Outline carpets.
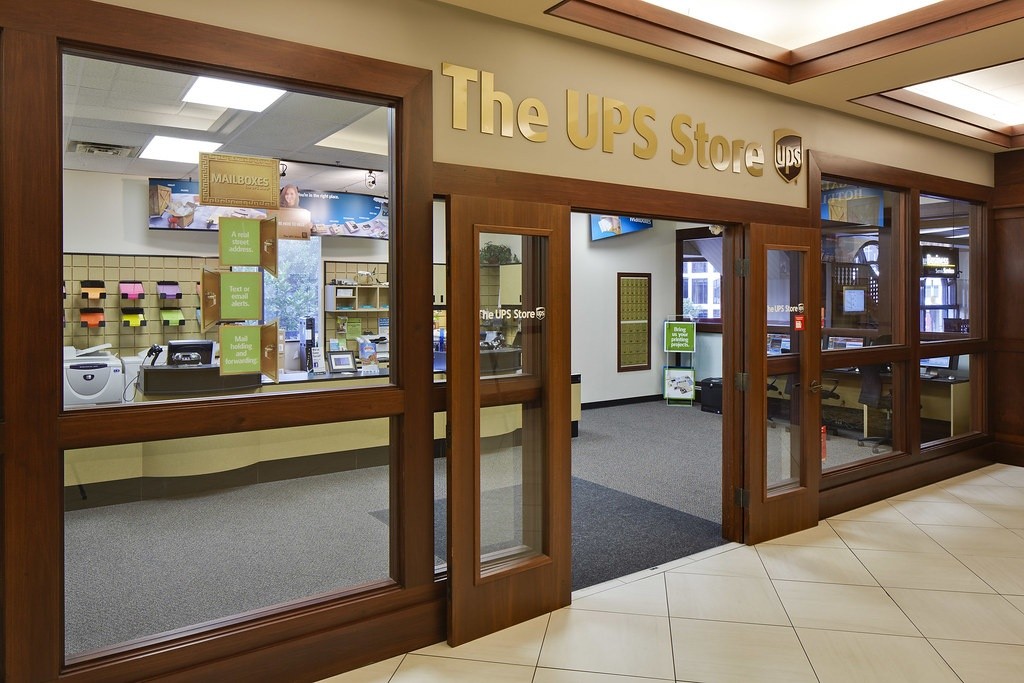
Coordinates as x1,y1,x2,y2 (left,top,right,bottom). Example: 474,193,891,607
368,476,731,593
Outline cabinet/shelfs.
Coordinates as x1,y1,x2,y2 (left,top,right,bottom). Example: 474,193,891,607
325,285,389,312
499,265,524,306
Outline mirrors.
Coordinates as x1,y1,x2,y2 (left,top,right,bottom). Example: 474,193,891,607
617,273,651,372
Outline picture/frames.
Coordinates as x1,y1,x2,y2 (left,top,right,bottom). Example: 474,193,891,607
327,351,357,373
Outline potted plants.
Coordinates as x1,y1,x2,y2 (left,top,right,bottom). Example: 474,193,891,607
479,239,520,265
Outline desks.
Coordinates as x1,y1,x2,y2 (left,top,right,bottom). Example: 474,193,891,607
864,373,970,438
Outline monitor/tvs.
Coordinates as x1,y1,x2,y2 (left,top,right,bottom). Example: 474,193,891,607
167,341,213,365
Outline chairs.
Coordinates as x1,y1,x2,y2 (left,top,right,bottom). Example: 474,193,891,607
781,349,841,436
767,375,779,427
858,366,893,453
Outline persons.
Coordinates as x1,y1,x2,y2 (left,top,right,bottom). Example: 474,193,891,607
279,184,300,208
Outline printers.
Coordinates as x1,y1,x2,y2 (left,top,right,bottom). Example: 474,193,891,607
121,346,167,402
64,343,122,407
